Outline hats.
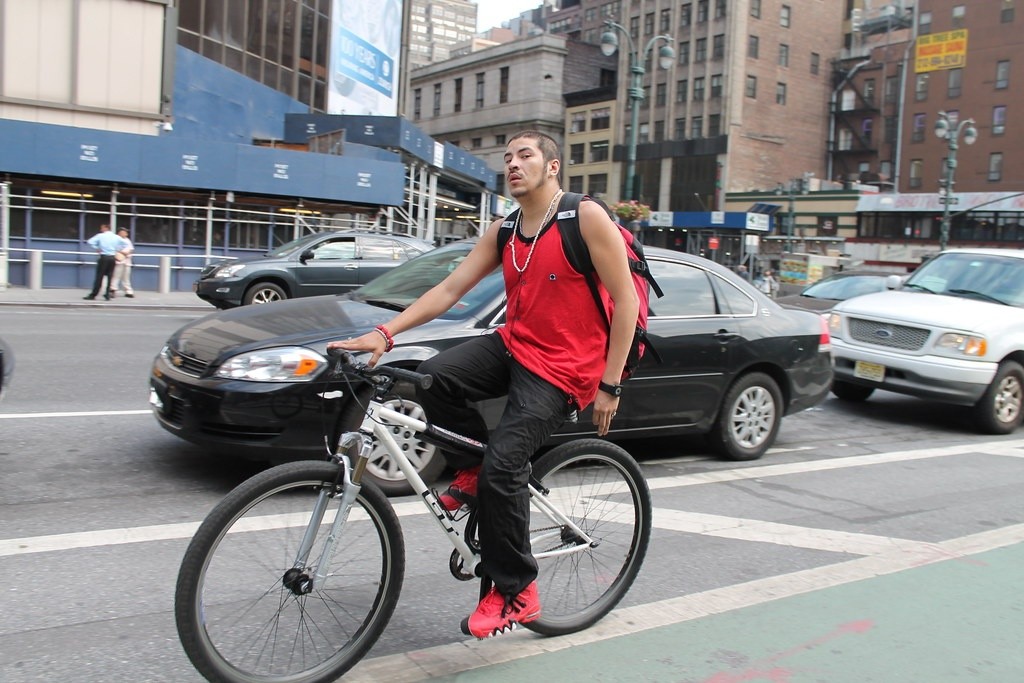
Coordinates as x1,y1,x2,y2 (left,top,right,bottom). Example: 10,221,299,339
116,227,130,233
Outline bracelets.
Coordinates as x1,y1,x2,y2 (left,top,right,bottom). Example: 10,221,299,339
373,325,394,353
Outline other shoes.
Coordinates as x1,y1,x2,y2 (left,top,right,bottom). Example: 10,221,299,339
109,290,116,298
125,294,134,298
104,294,110,300
83,294,95,300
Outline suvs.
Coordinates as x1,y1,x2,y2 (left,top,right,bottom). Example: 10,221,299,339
192,228,436,310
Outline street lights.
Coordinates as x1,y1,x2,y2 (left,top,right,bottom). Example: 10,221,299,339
773,177,810,253
934,110,978,251
600,19,677,203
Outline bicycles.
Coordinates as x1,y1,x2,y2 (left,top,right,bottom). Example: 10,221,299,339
175,348,652,683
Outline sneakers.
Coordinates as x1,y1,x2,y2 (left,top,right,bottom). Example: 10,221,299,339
460,578,543,640
435,463,483,511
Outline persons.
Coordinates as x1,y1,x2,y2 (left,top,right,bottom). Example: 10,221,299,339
324,131,640,638
756,270,776,296
83,224,125,299
109,227,134,298
736,264,750,282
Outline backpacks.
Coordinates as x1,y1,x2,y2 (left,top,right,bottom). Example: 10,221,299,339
495,191,665,381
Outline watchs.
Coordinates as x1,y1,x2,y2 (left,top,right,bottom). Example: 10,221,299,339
598,380,625,397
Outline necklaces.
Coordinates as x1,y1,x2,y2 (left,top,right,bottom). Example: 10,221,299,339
509,188,562,272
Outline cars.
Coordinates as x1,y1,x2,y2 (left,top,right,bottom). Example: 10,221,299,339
773,270,912,322
149,237,837,496
827,249,1024,435
